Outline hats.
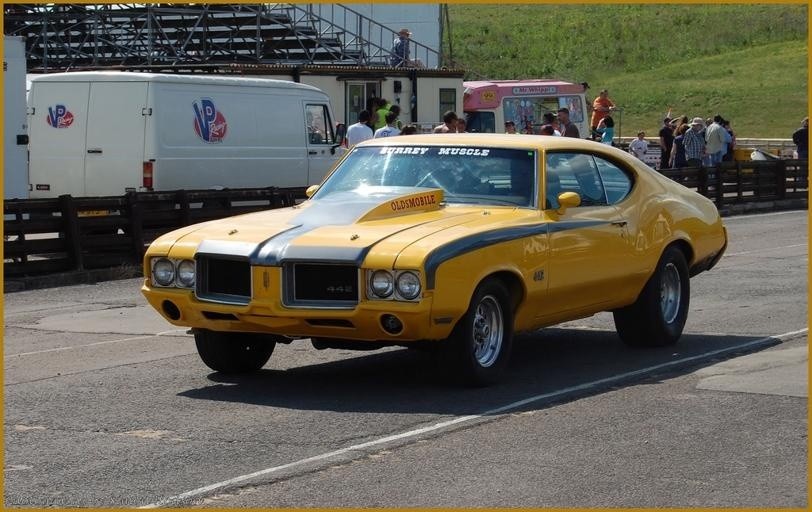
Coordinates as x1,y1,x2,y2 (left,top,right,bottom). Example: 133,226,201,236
687,117,705,127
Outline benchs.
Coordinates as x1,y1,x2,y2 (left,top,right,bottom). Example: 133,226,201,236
3,2,454,76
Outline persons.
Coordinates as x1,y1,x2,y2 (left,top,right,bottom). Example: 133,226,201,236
791,116,808,181
389,28,426,69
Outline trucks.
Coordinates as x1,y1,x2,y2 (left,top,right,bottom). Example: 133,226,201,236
30,67,359,209
461,75,593,146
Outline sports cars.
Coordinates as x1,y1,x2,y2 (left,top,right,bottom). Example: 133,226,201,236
142,132,728,387
